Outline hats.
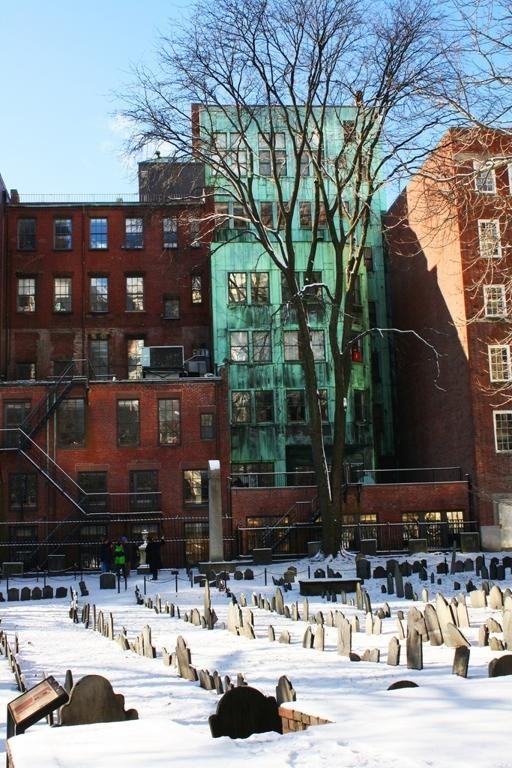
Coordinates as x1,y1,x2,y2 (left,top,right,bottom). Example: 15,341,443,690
121,536,127,542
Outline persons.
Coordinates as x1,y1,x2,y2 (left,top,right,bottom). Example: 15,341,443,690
144,535,164,581
98,536,138,579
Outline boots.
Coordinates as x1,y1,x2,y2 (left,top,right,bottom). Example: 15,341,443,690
150,570,157,580
